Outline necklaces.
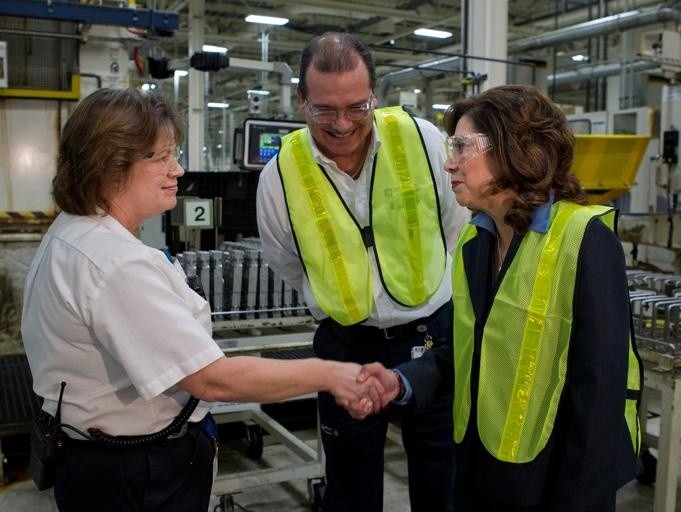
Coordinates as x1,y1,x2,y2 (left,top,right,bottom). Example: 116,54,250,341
497,235,504,272
352,135,371,180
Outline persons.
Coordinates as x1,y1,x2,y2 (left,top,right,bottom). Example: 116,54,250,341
251,31,477,509
333,83,648,509
15,83,390,510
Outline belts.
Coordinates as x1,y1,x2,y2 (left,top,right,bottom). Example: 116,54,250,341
384,301,450,340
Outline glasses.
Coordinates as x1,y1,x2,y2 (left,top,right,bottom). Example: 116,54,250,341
444,132,497,169
142,144,181,171
303,89,375,124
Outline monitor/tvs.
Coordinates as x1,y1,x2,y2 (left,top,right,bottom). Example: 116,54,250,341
242,119,307,172
